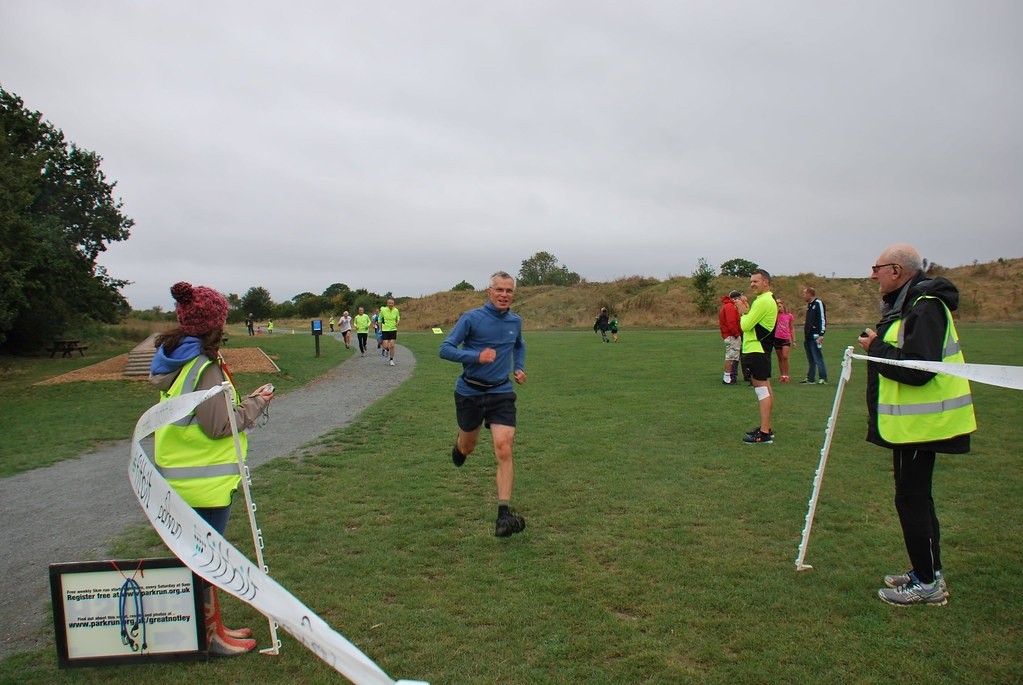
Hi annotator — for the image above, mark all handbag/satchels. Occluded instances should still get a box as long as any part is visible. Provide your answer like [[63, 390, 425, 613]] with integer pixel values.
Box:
[[461, 372, 508, 392], [607, 324, 612, 330], [594, 323, 600, 333]]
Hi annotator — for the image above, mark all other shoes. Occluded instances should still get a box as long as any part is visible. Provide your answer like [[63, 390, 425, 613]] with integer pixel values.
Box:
[[744, 377, 752, 381], [731, 378, 736, 382], [722, 380, 735, 385]]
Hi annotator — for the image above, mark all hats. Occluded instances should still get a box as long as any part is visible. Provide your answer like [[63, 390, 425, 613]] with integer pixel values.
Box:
[[729, 290, 743, 297], [170, 282, 229, 336]]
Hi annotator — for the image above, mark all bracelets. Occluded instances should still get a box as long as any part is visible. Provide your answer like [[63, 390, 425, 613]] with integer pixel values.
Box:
[[792, 340, 796, 342]]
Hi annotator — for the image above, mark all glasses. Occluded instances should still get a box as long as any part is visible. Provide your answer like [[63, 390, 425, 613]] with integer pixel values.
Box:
[[871, 264, 902, 273]]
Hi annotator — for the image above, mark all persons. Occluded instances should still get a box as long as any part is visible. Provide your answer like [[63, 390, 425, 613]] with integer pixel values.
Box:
[[337, 310, 352, 349], [857, 246, 977, 606], [372, 308, 389, 357], [353, 306, 371, 357], [609, 314, 618, 342], [257, 327, 262, 336], [266, 318, 273, 336], [717, 290, 751, 385], [437, 270, 527, 538], [147, 283, 273, 655], [595, 306, 609, 342], [328, 314, 335, 333], [798, 287, 829, 384], [773, 298, 797, 383], [739, 270, 778, 443], [377, 298, 400, 366], [245, 313, 255, 336]]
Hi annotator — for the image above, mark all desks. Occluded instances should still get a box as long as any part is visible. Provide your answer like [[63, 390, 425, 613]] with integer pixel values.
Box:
[[50, 341, 84, 358]]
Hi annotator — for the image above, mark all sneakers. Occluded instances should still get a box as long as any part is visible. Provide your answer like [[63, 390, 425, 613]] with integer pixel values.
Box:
[[799, 378, 816, 384], [741, 433, 773, 444], [452, 434, 466, 467], [382, 350, 385, 356], [385, 350, 389, 357], [745, 428, 775, 437], [885, 569, 950, 598], [818, 378, 828, 384], [778, 377, 784, 382], [784, 377, 790, 382], [495, 513, 526, 537], [878, 580, 949, 605], [389, 360, 395, 365]]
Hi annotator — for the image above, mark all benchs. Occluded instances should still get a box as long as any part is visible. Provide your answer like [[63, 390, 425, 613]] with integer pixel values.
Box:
[[47, 347, 88, 352]]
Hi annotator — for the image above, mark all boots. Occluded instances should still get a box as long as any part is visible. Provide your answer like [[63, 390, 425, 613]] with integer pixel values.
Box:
[[201, 586, 257, 655], [223, 625, 253, 639]]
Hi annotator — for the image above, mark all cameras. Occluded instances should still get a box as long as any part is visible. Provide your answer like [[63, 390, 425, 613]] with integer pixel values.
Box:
[[264, 386, 276, 393]]
